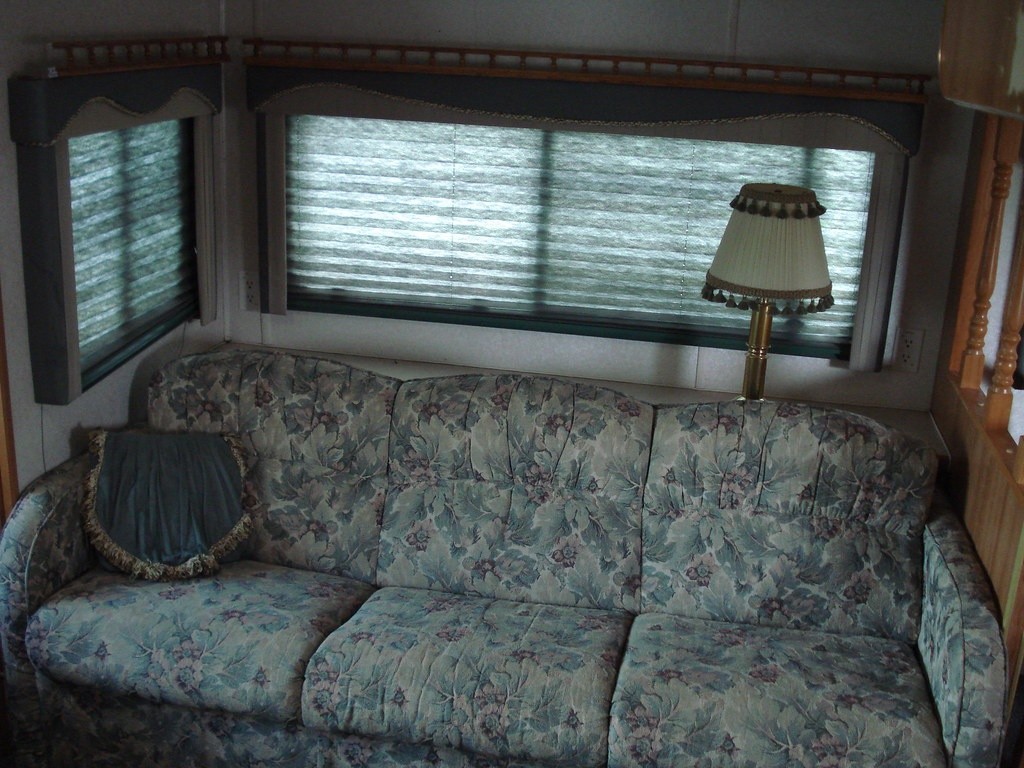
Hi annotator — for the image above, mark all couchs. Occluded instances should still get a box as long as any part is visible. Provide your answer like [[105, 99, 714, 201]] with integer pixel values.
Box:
[[0, 347, 1007, 768]]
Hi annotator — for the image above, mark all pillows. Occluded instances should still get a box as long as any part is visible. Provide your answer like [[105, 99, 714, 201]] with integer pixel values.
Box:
[[82, 429, 260, 582]]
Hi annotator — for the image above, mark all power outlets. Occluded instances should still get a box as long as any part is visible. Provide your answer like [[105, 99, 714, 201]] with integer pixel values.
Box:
[[890, 328, 924, 374]]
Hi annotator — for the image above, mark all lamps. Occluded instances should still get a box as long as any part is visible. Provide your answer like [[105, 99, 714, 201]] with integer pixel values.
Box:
[[702, 184, 833, 400]]
